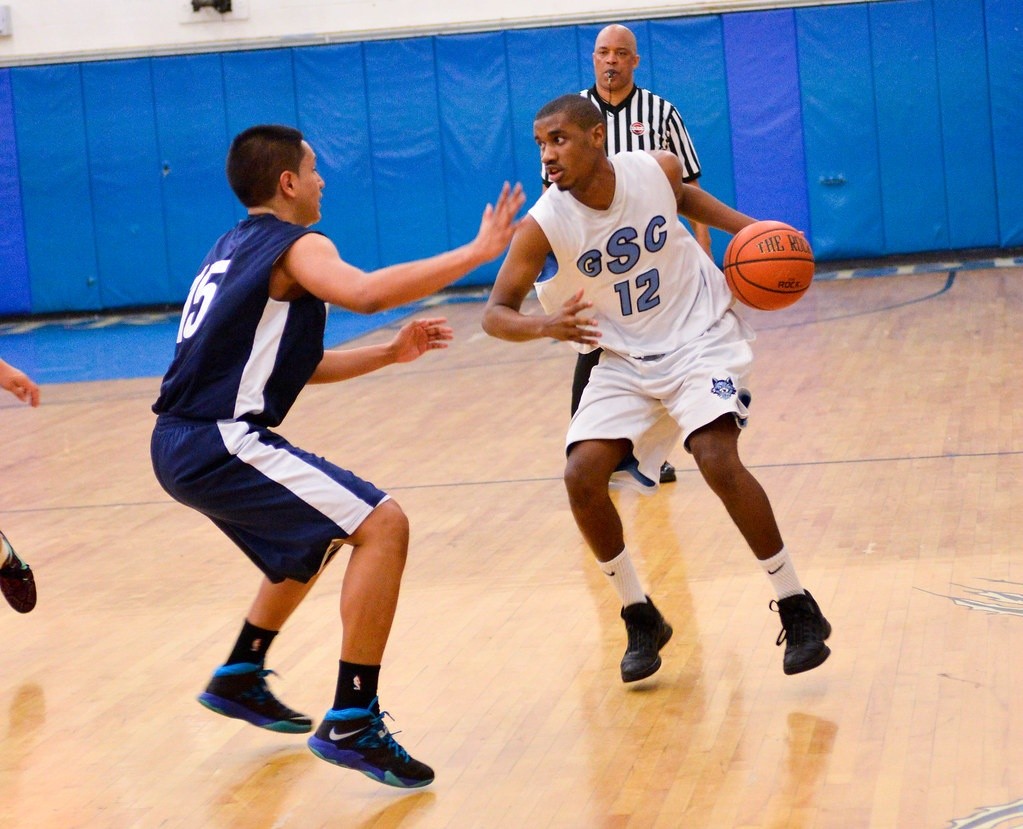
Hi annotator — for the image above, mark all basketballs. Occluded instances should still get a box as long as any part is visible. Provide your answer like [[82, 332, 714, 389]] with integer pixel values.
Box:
[[723, 220, 814, 311]]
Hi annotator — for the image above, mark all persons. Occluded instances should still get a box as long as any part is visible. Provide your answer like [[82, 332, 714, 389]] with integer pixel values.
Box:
[[481, 93, 833, 684], [154, 126, 524, 793], [0, 356, 40, 614], [540, 22, 715, 486]]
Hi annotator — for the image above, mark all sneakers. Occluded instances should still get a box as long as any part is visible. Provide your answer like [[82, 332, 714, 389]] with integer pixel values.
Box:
[[0, 531, 37, 614], [777, 589, 832, 674], [307, 696, 435, 788], [620, 595, 672, 683], [197, 658, 312, 735]]
